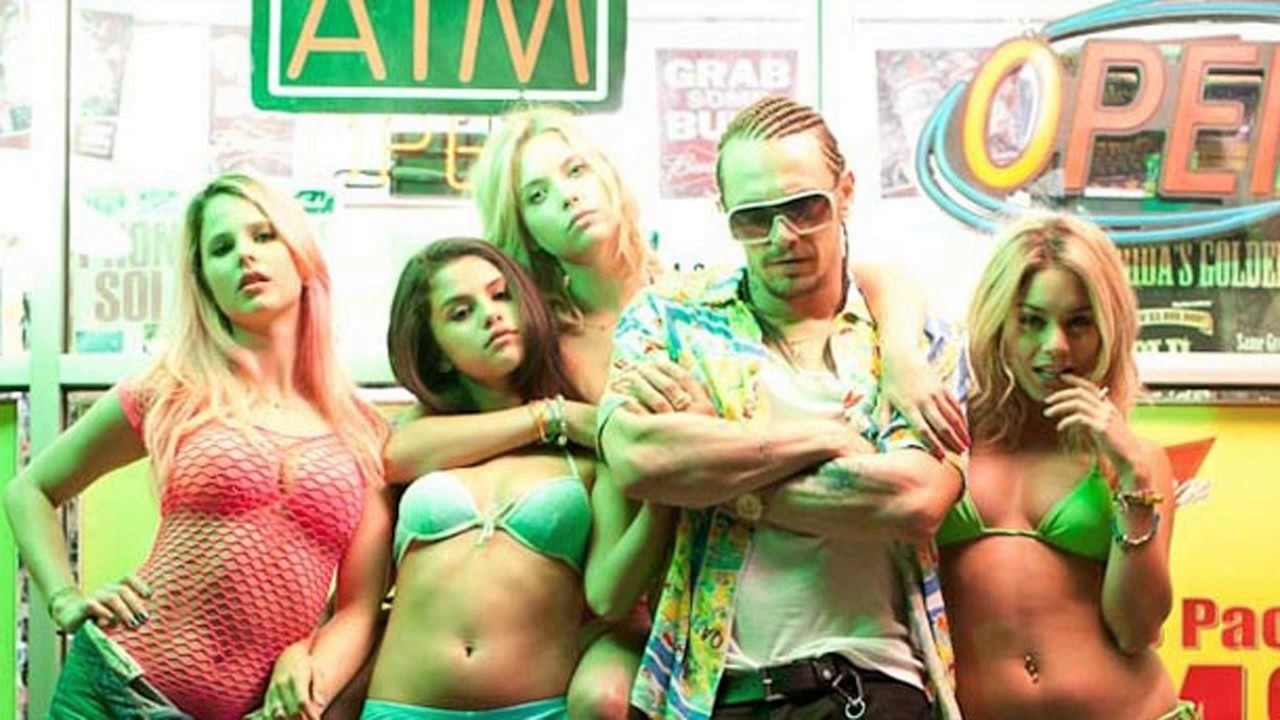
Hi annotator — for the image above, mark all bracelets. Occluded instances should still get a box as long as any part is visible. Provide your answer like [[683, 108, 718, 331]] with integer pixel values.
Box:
[[553, 394, 569, 446], [1115, 489, 1164, 509], [47, 585, 82, 618], [537, 398, 558, 442], [1112, 513, 1162, 549], [526, 399, 545, 443]]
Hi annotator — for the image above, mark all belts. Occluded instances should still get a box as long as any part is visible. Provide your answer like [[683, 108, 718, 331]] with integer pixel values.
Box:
[[717, 653, 884, 706]]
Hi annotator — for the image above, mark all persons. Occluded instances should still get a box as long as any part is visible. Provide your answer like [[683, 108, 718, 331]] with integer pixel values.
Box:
[[263, 98, 1197, 720], [2, 173, 402, 720]]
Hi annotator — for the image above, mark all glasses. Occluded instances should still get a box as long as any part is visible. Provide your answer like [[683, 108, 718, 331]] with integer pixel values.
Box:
[[729, 188, 835, 244]]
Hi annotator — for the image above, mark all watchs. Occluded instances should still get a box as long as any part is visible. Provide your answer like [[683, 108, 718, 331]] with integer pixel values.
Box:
[[737, 492, 767, 530]]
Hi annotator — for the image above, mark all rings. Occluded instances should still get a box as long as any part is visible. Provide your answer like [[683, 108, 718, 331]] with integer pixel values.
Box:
[[1098, 386, 1107, 398]]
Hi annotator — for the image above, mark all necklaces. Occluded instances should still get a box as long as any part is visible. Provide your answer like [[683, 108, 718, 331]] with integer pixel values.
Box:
[[787, 325, 823, 361], [250, 383, 294, 411], [586, 320, 617, 333]]
